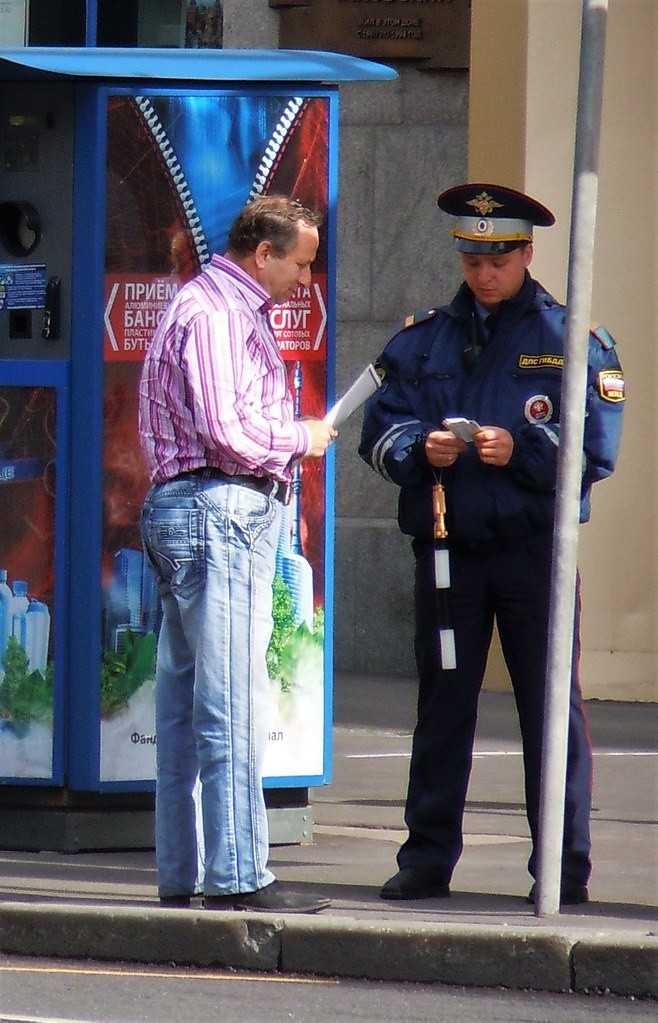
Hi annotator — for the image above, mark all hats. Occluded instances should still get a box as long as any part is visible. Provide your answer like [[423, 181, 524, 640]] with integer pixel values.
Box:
[[438, 183, 556, 255]]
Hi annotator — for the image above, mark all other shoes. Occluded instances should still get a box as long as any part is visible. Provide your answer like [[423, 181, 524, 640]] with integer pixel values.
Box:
[[528, 883, 588, 904], [380, 869, 450, 900]]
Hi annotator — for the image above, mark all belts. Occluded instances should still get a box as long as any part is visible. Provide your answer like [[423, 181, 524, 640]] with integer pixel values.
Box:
[[181, 466, 292, 506]]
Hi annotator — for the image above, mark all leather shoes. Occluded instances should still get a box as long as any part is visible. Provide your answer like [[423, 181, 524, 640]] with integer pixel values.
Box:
[[206, 880, 331, 912], [159, 891, 206, 908]]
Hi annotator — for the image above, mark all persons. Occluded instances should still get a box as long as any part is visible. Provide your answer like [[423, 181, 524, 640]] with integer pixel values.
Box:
[[357, 181, 626, 906], [139, 195, 337, 914]]
